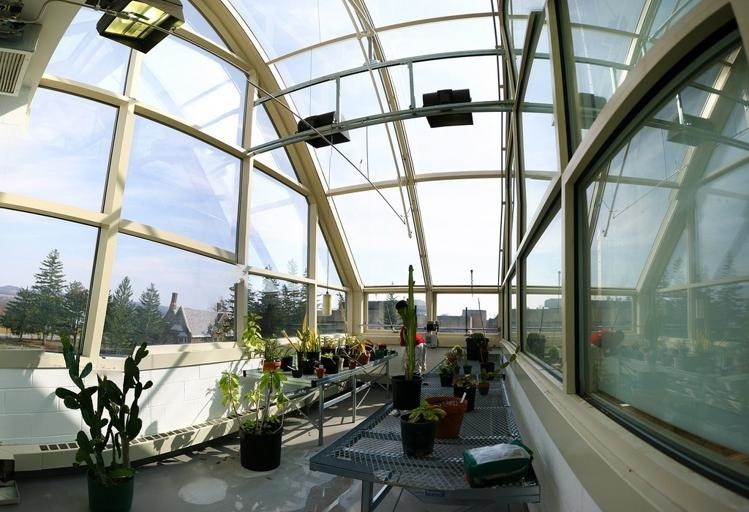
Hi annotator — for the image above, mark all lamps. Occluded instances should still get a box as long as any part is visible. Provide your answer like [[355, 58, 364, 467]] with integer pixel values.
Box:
[[423, 90, 474, 128], [297, 111, 351, 148], [322, 204, 332, 318], [96, 0, 185, 54]]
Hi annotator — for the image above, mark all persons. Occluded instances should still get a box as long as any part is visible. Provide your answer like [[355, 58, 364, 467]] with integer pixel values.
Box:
[[400, 326, 408, 373], [413, 332, 428, 376], [591, 326, 626, 359]]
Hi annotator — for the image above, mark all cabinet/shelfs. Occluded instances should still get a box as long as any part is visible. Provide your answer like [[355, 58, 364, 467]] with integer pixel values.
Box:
[[310, 352, 541, 512]]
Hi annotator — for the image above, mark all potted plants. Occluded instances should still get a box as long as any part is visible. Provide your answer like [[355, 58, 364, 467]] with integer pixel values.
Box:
[[669, 340, 691, 369], [240, 311, 388, 380], [390, 264, 424, 410], [526, 333, 560, 359], [441, 333, 497, 411], [219, 368, 289, 472], [56, 334, 154, 512], [426, 395, 468, 440], [399, 402, 446, 458]]
